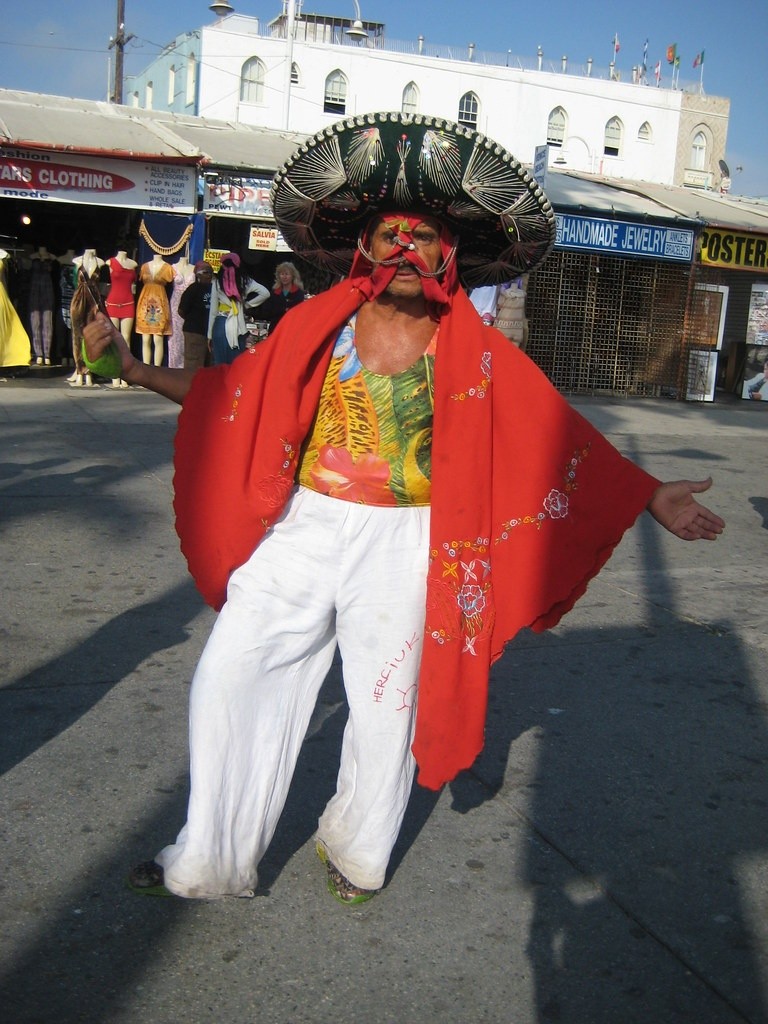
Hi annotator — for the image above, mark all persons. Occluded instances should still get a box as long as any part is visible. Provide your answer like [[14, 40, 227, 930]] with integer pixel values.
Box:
[[742, 361, 768, 401], [68, 249, 196, 388], [0, 246, 79, 368], [83, 206, 726, 905], [268, 262, 305, 336], [493, 283, 528, 346], [178, 261, 213, 371], [207, 253, 270, 367]]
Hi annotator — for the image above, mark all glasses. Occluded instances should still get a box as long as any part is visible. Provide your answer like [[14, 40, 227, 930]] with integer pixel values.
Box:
[[197, 270, 214, 275]]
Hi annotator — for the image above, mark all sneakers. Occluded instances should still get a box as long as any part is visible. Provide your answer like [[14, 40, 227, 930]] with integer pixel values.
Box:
[[316, 834, 374, 904], [129, 860, 178, 897]]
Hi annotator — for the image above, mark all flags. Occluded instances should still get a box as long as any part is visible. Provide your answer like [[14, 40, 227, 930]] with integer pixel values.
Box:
[[611, 33, 704, 81]]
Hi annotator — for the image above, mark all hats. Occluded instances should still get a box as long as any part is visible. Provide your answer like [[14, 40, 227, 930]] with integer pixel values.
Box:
[[482, 313, 495, 322], [268, 110, 557, 290]]
